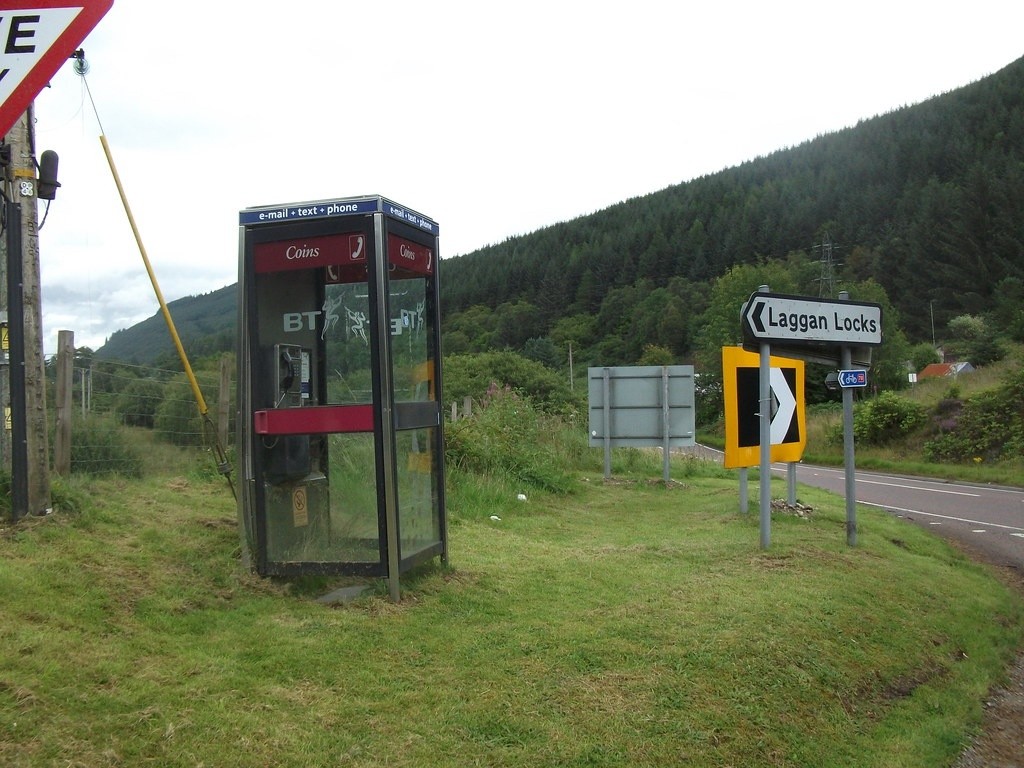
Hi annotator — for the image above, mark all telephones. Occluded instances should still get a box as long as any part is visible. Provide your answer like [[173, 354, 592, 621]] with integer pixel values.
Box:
[[272, 342, 303, 408]]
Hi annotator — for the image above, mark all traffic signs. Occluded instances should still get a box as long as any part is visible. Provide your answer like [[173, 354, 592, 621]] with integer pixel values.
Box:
[[838, 368, 868, 387], [742, 291, 885, 346]]
